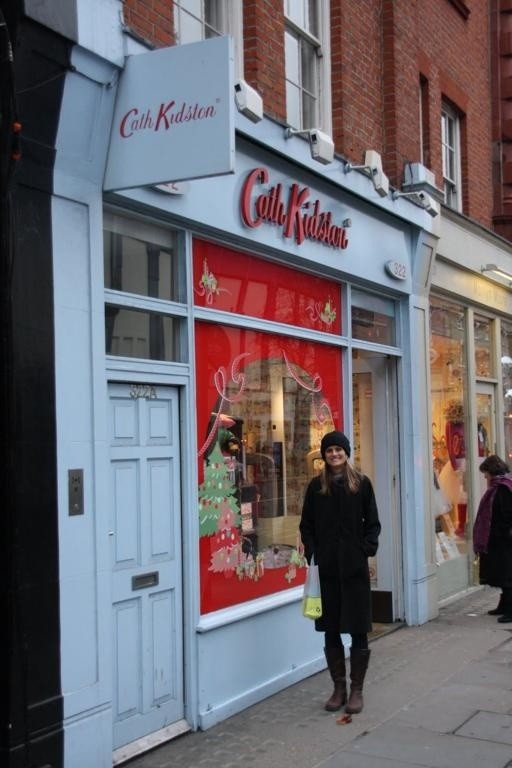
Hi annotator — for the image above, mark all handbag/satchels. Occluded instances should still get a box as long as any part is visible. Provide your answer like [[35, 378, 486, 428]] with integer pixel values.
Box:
[[303, 565, 323, 619]]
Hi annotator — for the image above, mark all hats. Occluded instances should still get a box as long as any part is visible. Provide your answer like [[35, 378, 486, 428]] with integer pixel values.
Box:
[[321, 431, 350, 458]]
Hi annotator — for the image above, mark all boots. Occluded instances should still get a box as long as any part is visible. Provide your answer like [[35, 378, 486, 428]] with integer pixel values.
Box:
[[346, 648, 370, 713], [488, 594, 511, 622], [323, 646, 346, 711]]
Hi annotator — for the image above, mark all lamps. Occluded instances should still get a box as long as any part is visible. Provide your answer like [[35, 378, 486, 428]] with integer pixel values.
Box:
[[234, 80, 264, 124], [481, 264, 512, 287], [344, 162, 389, 197], [284, 127, 335, 165], [392, 189, 438, 217]]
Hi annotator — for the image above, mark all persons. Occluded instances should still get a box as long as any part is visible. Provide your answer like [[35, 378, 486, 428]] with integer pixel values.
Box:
[[473, 455, 512, 623], [445, 395, 484, 538], [299, 431, 380, 714]]
